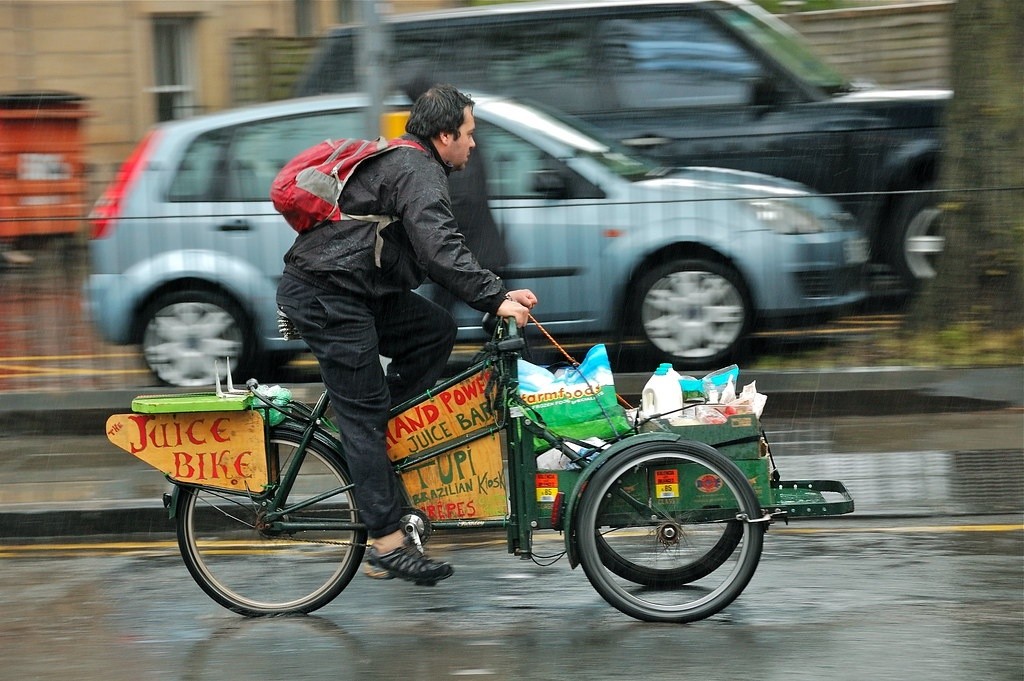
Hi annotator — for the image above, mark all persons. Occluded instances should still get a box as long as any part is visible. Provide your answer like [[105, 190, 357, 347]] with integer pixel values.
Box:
[[407, 78, 507, 388], [275, 85, 537, 582]]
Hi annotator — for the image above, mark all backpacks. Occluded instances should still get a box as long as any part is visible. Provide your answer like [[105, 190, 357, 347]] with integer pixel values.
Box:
[[271, 136, 429, 268]]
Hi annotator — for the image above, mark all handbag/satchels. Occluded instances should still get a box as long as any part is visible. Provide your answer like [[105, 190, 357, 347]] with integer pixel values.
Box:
[[517, 344, 636, 451], [678, 363, 739, 402]]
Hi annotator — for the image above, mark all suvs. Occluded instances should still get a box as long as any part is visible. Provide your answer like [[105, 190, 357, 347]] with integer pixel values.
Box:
[[79, 86, 864, 399], [246, 2, 955, 304]]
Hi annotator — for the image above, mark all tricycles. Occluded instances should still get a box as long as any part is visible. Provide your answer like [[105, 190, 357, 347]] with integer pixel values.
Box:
[[106, 299, 851, 626]]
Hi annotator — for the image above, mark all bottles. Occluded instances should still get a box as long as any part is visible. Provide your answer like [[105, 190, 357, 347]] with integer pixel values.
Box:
[[660, 363, 681, 379], [656, 367, 683, 404], [642, 371, 683, 421]]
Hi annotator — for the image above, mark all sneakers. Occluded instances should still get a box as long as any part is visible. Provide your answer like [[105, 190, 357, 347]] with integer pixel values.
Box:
[[363, 534, 454, 581]]
